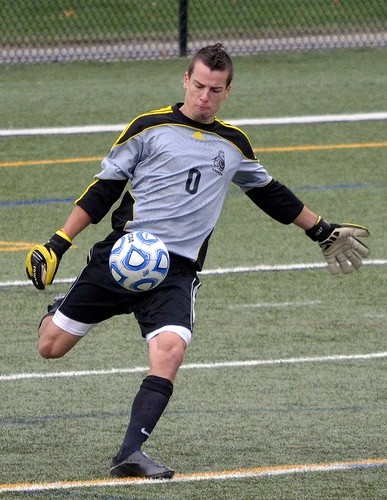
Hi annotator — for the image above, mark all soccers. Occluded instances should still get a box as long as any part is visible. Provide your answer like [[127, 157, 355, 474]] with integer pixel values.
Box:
[[109, 231, 169, 291]]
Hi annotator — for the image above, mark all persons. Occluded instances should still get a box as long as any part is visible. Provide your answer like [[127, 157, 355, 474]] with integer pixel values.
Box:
[[25, 42, 370, 479]]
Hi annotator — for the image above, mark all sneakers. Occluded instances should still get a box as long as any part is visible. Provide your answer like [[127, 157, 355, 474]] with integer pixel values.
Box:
[[110, 451, 176, 479], [47, 292, 68, 312]]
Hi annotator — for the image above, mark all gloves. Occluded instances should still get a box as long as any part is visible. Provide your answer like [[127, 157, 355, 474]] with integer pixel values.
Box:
[[306, 216, 371, 277], [25, 231, 74, 290]]
